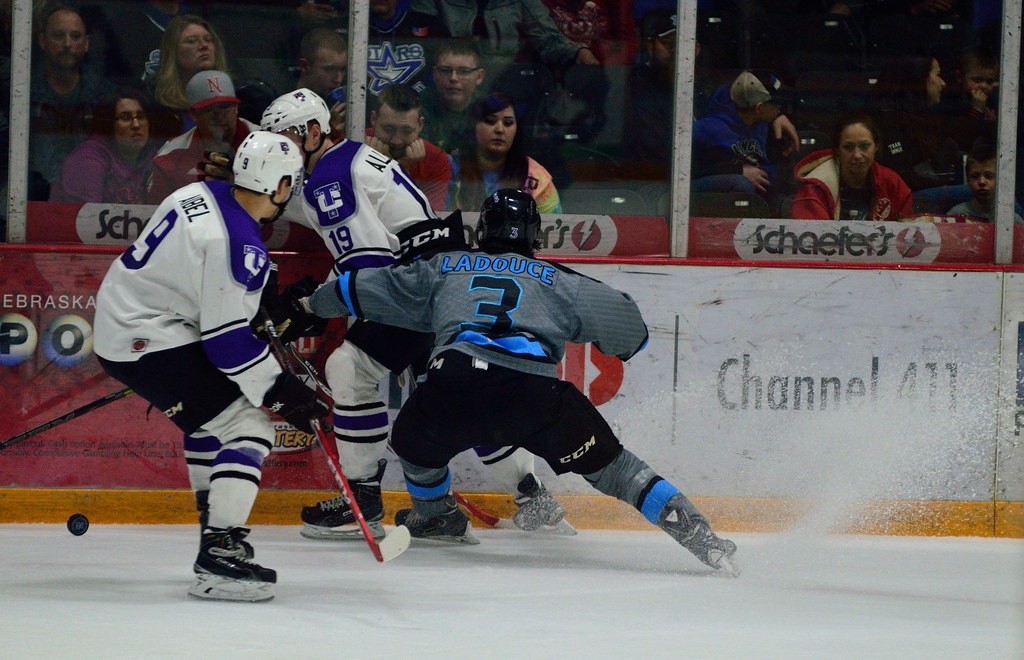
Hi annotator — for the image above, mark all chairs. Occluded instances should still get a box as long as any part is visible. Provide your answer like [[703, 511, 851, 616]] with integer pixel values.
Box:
[[554, 39, 972, 217]]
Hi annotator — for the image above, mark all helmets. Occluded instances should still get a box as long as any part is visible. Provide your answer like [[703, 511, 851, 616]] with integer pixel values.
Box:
[[474, 187, 541, 248], [260, 87, 332, 135], [232, 131, 305, 196]]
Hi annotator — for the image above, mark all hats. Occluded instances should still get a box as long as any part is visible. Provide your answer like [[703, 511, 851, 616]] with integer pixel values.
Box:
[[730, 68, 793, 107], [640, 7, 677, 41], [185, 70, 241, 112]]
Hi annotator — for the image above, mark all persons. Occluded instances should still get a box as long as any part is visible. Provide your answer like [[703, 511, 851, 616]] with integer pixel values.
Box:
[[92, 132, 334, 603], [0, 0, 1024, 225], [260, 89, 576, 537], [295, 190, 736, 578]]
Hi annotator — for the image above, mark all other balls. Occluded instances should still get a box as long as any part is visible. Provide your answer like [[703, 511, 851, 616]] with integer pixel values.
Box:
[[66, 513, 89, 536]]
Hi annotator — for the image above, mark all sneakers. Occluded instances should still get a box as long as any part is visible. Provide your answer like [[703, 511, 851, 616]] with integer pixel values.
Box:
[[658, 492, 740, 577], [188, 526, 278, 601], [513, 473, 578, 536], [299, 459, 388, 541], [196, 489, 254, 560], [395, 484, 482, 545]]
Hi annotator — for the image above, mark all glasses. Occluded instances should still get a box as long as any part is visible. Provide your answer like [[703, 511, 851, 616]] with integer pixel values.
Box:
[[434, 65, 479, 75], [111, 111, 147, 123]]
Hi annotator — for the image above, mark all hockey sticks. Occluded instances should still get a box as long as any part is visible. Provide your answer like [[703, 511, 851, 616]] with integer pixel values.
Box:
[[261, 311, 413, 565], [0, 387, 136, 451], [284, 341, 578, 534]]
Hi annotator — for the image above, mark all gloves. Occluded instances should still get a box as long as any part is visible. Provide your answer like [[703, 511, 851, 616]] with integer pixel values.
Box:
[[262, 373, 330, 434], [196, 150, 234, 185], [251, 274, 329, 352]]
